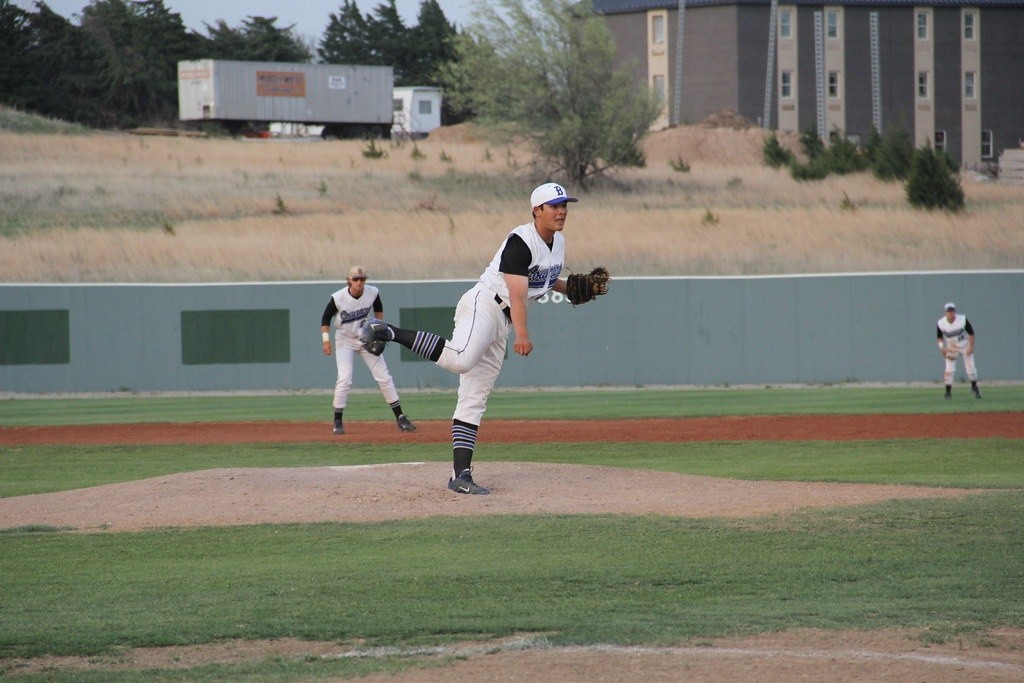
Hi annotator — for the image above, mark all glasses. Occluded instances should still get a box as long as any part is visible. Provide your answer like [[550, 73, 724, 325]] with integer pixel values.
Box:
[[350, 277, 367, 281]]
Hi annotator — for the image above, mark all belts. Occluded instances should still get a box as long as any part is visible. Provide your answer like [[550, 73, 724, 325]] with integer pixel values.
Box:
[[494, 293, 514, 320]]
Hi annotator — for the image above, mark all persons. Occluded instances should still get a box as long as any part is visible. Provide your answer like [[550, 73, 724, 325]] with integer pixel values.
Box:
[[321, 265, 416, 435], [361, 183, 610, 495], [936, 302, 982, 398]]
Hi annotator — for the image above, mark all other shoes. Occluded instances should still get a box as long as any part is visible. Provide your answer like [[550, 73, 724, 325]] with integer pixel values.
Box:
[[945, 391, 951, 401], [972, 386, 982, 399]]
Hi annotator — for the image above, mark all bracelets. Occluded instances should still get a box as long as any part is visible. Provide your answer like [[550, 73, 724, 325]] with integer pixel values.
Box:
[[938, 342, 943, 349], [322, 332, 330, 344]]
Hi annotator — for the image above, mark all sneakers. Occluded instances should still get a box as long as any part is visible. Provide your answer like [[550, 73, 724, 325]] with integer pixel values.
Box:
[[334, 420, 345, 436], [447, 466, 491, 495], [397, 416, 417, 432], [359, 317, 387, 343]]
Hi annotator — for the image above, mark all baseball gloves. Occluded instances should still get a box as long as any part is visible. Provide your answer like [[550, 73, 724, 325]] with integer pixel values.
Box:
[[943, 348, 958, 360], [363, 340, 390, 356], [565, 266, 612, 309]]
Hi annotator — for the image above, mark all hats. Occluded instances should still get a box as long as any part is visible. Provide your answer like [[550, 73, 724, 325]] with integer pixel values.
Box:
[[349, 264, 369, 279], [944, 303, 955, 312], [530, 180, 578, 209]]
